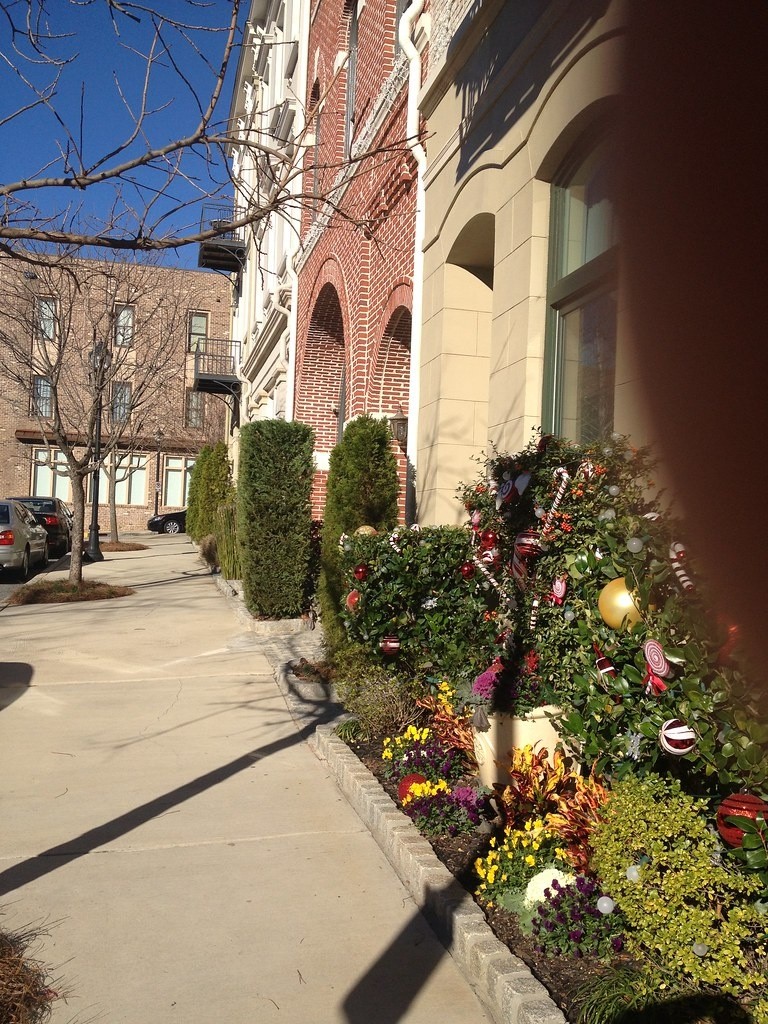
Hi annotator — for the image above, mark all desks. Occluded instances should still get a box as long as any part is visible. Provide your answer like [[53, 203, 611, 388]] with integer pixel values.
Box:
[[209, 220, 231, 239]]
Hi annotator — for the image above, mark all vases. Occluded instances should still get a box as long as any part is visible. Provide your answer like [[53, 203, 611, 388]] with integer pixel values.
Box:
[[471, 700, 581, 797]]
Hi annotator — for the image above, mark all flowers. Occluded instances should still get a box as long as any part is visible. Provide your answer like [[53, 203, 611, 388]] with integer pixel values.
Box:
[[460, 425, 620, 717]]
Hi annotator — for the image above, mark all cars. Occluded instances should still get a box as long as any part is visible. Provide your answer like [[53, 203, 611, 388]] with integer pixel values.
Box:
[[0, 499, 50, 579], [147, 508, 188, 535], [5, 494, 75, 559]]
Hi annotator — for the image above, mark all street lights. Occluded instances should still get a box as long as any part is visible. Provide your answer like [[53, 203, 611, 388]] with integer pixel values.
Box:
[[79, 339, 105, 563]]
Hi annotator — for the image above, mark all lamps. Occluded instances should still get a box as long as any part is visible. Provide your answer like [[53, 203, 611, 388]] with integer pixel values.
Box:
[[387, 404, 408, 459]]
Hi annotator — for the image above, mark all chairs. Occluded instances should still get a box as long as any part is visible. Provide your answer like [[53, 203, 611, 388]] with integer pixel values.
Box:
[[225, 230, 238, 241], [28, 504, 55, 512]]
